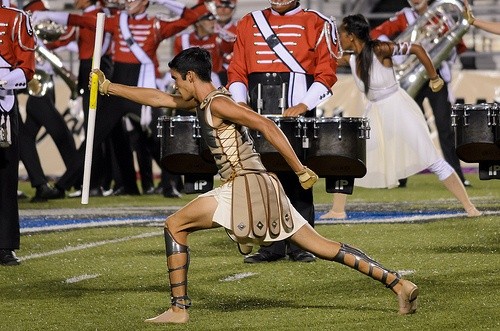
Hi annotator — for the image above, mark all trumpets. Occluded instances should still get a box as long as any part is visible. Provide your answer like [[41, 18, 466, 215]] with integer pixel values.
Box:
[[33, 45, 82, 100], [27, 69, 53, 98]]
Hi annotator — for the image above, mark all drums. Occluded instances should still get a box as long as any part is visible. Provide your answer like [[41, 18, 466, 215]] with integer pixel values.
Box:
[[247, 114, 295, 169], [156, 115, 218, 176], [295, 114, 370, 179], [450, 103, 500, 163]]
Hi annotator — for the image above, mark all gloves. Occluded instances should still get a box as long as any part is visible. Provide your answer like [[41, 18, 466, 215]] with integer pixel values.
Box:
[[31, 11, 42, 26], [88, 68, 110, 96], [150, 0, 167, 6], [429, 75, 444, 92], [295, 166, 319, 189]]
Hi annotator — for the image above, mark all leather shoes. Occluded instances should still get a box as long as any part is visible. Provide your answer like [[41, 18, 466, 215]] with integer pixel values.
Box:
[[0, 249, 20, 266], [287, 252, 316, 262], [244, 250, 286, 264]]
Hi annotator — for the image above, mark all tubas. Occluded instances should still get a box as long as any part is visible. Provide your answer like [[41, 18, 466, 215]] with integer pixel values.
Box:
[[392, 0, 471, 99]]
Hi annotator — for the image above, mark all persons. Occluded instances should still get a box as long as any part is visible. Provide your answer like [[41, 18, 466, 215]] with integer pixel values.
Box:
[[319, 12, 484, 219], [464, 0, 500, 35], [369, 0, 472, 187], [0, 0, 239, 202], [88, 47, 419, 323], [0, 0, 39, 266], [226, 0, 338, 263]]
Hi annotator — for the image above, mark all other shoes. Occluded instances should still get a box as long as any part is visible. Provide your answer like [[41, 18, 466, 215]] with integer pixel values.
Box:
[[398, 280, 418, 314], [145, 308, 189, 323], [467, 207, 482, 216], [319, 211, 346, 219]]
